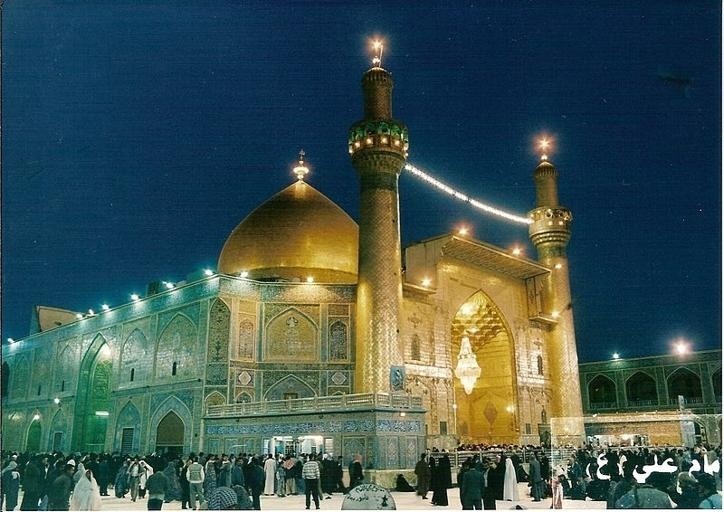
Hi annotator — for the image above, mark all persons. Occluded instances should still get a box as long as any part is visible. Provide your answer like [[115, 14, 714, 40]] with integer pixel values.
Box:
[[415, 446, 452, 506], [0, 444, 365, 510], [456, 434, 722, 509]]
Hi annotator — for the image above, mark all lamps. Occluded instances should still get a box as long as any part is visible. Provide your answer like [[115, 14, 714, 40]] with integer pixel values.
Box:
[[454, 329, 482, 396]]
